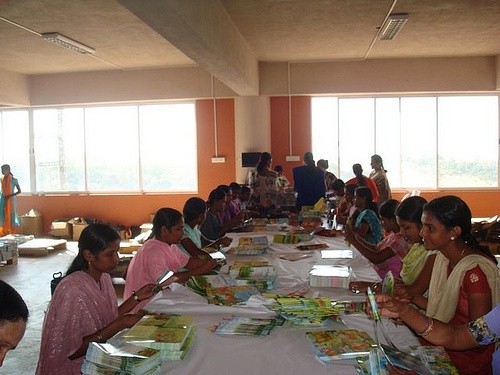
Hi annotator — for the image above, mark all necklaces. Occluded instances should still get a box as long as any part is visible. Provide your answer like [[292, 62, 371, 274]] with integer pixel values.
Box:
[[459, 246, 467, 259]]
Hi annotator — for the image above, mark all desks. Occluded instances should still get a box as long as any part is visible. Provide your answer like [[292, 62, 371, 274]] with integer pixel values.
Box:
[[143, 224, 423, 375]]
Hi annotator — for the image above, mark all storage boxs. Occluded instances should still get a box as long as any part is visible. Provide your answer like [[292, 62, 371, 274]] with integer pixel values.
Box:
[[50, 218, 89, 240], [20, 214, 44, 236]]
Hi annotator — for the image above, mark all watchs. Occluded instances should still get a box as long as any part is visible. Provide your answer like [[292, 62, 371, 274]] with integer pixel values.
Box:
[[132, 291, 141, 303]]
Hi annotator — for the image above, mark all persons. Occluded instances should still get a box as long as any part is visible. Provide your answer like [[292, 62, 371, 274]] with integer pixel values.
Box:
[[176, 198, 233, 261], [199, 180, 254, 246], [248, 152, 283, 220], [0, 277, 29, 373], [122, 205, 218, 315], [0, 163, 23, 236], [35, 223, 166, 374], [292, 151, 500, 375], [274, 166, 291, 193]]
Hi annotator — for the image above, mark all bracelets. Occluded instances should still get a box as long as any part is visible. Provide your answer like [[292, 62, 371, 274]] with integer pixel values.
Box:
[[416, 317, 434, 337]]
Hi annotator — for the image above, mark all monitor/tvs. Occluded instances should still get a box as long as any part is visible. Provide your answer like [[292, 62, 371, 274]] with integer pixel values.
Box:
[[242, 153, 261, 167]]
